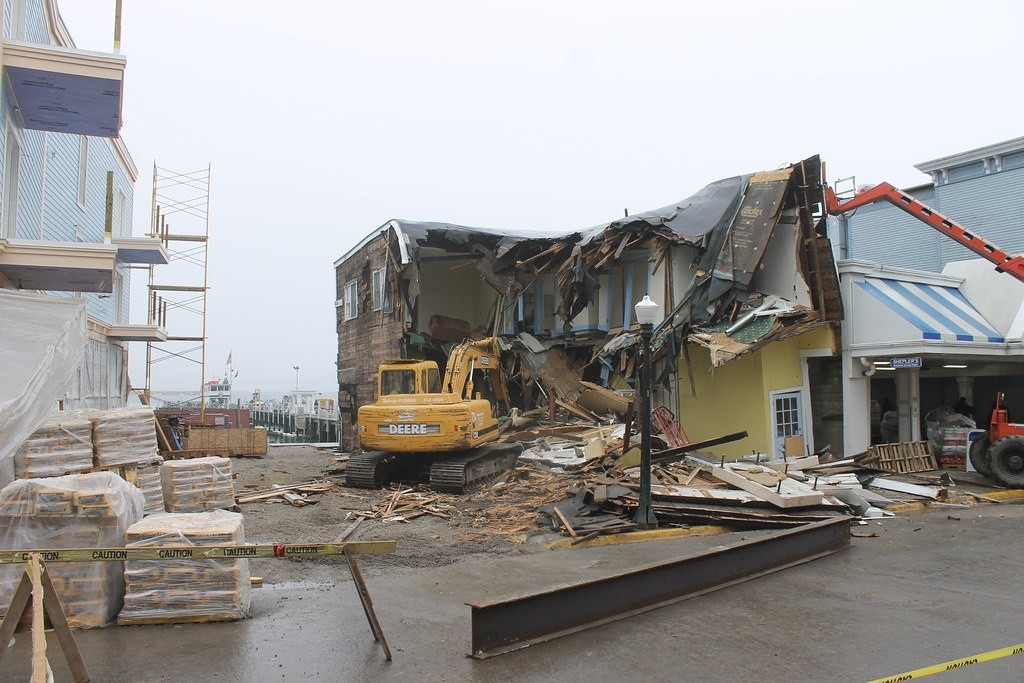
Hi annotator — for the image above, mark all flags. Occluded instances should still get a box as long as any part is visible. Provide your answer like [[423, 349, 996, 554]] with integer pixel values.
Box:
[[227, 353, 231, 365]]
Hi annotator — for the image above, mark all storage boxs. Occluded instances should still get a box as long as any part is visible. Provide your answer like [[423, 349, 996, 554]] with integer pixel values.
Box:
[[784, 433, 805, 457], [428, 314, 472, 343], [579, 380, 634, 420]]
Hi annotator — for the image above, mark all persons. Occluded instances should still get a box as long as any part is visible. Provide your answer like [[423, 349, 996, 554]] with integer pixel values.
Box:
[[314, 400, 318, 414], [954, 397, 975, 417]]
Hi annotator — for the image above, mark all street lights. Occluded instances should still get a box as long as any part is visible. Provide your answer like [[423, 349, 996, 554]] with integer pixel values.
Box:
[[633, 295, 659, 528]]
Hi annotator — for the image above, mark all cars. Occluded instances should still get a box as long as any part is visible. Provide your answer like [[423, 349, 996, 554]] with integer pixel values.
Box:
[[249, 399, 268, 410], [265, 400, 274, 408]]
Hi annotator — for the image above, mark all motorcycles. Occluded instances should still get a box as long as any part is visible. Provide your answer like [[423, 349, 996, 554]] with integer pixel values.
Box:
[[314, 398, 334, 415]]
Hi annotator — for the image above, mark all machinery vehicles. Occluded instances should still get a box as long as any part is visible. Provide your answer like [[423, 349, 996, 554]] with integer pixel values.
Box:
[[345, 336, 524, 495], [826, 183, 1024, 489]]
